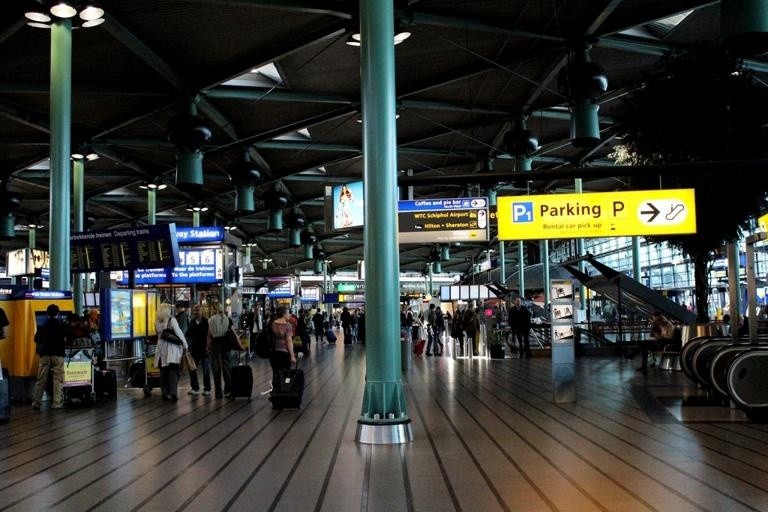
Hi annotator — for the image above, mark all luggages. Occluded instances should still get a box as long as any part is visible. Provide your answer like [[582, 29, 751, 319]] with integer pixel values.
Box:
[[413, 337, 426, 354], [233, 348, 252, 397], [325, 330, 336, 342], [272, 360, 304, 409], [0, 368, 11, 419], [344, 334, 353, 344]]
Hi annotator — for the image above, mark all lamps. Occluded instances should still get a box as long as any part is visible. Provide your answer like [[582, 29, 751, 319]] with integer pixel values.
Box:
[[258, 256, 273, 263], [345, 27, 411, 47], [138, 180, 168, 190], [186, 202, 209, 213], [242, 239, 258, 247], [21, 220, 44, 229], [224, 224, 237, 231], [70, 150, 100, 162], [24, 0, 106, 29]]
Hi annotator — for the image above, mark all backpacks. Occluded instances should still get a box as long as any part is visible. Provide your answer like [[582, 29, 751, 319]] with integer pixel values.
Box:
[[255, 318, 275, 359]]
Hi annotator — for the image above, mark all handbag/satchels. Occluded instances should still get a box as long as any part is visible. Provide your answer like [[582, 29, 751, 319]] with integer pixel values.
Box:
[[183, 348, 196, 371], [160, 316, 183, 345]]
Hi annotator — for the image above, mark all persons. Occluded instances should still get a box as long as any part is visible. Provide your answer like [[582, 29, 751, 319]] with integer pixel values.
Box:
[[242, 297, 532, 359], [153, 302, 188, 401], [175, 300, 192, 332], [267, 305, 297, 401], [206, 302, 233, 397], [718, 314, 731, 337], [185, 304, 212, 395]]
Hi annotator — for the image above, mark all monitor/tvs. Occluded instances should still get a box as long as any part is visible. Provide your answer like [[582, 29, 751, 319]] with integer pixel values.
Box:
[[399, 168, 414, 200], [36, 314, 70, 326], [70, 238, 171, 269], [324, 185, 363, 234], [332, 179, 366, 232]]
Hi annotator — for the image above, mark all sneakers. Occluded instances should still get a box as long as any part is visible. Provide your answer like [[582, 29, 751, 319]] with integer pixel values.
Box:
[[188, 389, 200, 395], [215, 392, 231, 399], [270, 391, 273, 395], [202, 391, 211, 395], [52, 402, 62, 408], [32, 403, 41, 409]]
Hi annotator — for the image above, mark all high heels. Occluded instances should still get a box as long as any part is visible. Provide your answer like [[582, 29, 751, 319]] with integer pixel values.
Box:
[[161, 394, 177, 402]]
